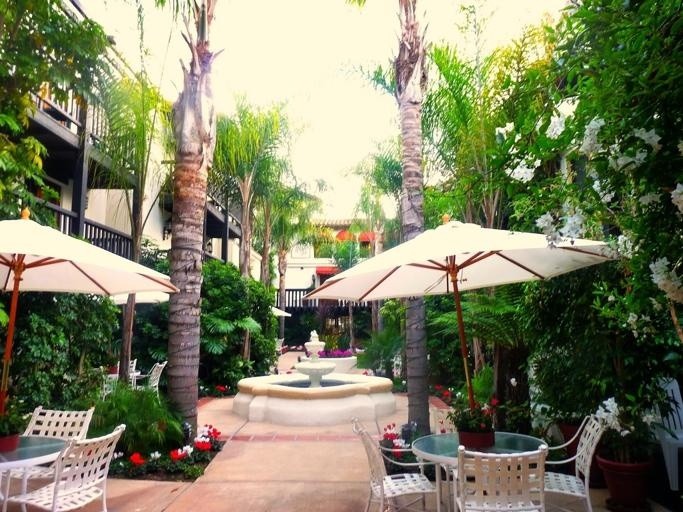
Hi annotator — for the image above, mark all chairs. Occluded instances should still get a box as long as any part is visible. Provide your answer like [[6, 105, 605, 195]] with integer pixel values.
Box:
[[0, 405, 127, 511], [273, 338, 287, 357], [637, 377, 683, 491], [351, 414, 609, 512], [391, 350, 403, 380], [98, 358, 170, 404]]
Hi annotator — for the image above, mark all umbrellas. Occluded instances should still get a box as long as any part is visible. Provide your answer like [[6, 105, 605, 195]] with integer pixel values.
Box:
[[268, 304, 290, 318], [0, 217, 178, 412], [52, 291, 171, 307], [302, 222, 620, 413]]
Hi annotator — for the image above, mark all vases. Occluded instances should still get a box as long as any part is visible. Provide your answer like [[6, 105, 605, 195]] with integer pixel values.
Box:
[[594, 447, 664, 511]]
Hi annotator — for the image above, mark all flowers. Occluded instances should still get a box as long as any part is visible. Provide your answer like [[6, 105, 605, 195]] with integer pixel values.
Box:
[[593, 374, 677, 458], [436, 387, 505, 432]]
